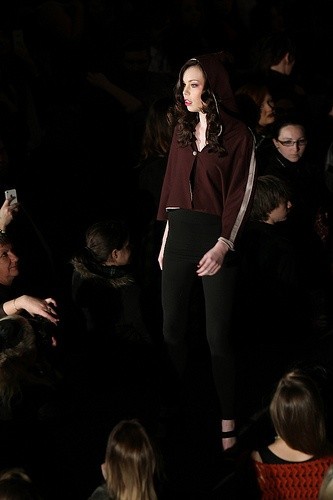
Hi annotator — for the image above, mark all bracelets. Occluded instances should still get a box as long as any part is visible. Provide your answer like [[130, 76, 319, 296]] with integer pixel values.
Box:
[[218, 236, 234, 249], [14, 299, 19, 311]]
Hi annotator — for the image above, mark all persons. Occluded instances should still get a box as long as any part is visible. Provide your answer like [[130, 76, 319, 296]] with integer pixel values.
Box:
[[156, 53, 261, 461], [70, 217, 152, 344], [0, 197, 59, 500], [87, 421, 157, 500], [251, 368, 333, 484], [0, 0, 333, 303]]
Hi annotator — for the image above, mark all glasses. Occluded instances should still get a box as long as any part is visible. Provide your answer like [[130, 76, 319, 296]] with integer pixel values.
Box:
[[275, 138, 307, 145]]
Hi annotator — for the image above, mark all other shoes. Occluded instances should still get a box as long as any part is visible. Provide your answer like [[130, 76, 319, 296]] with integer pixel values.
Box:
[[219, 419, 237, 455]]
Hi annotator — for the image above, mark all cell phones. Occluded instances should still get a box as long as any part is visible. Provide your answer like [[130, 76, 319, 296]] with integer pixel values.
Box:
[[5, 189, 18, 211]]
